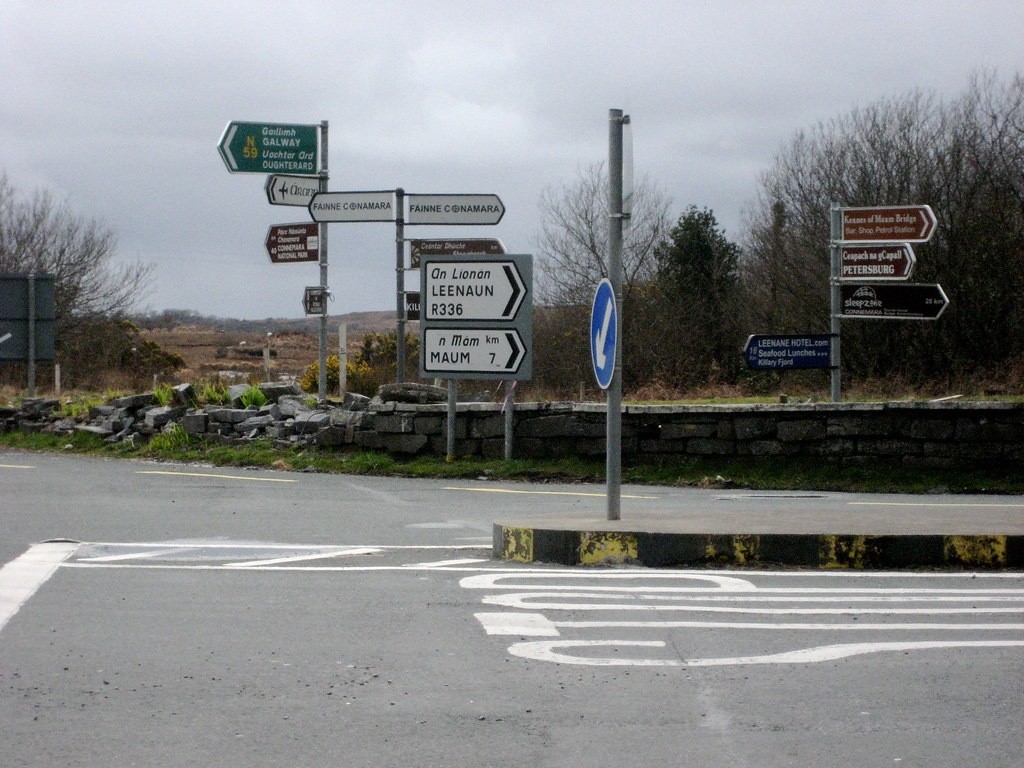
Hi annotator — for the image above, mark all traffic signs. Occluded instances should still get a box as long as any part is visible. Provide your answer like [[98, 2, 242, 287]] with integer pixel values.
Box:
[[737, 334, 830, 371], [263, 222, 322, 263], [840, 203, 937, 242], [217, 121, 321, 176], [840, 243, 917, 281], [423, 260, 529, 321], [423, 328, 527, 372], [839, 283, 950, 331], [263, 174, 323, 207]]
